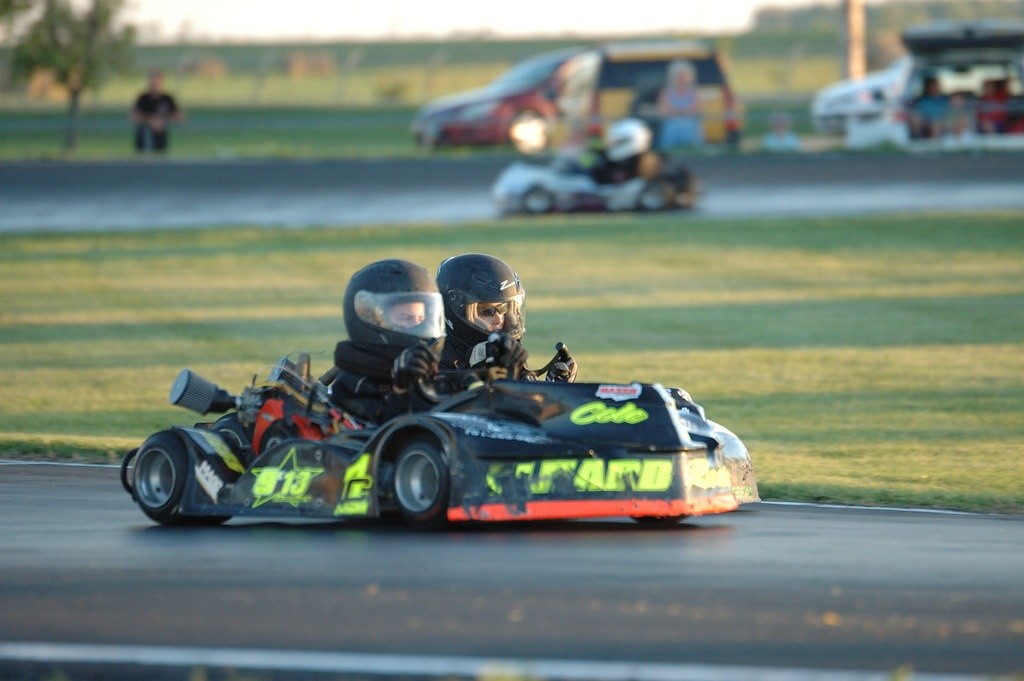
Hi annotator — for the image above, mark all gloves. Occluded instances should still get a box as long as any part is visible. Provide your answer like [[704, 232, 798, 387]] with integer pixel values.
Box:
[[484, 333, 528, 370], [391, 342, 437, 387], [545, 357, 577, 383]]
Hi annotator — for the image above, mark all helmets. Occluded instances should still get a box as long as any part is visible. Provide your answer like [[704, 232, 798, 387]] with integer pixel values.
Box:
[[436, 252, 525, 349], [603, 119, 650, 160], [343, 257, 446, 360]]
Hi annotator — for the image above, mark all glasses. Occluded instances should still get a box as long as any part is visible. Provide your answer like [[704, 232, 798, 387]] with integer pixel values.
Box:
[[477, 303, 508, 317]]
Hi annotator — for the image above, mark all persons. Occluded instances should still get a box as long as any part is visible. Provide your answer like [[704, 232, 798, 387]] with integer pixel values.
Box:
[[910, 78, 951, 137], [326, 256, 444, 427], [436, 254, 577, 383], [975, 78, 1008, 132], [657, 59, 704, 147], [130, 63, 186, 154], [580, 119, 658, 183]]
